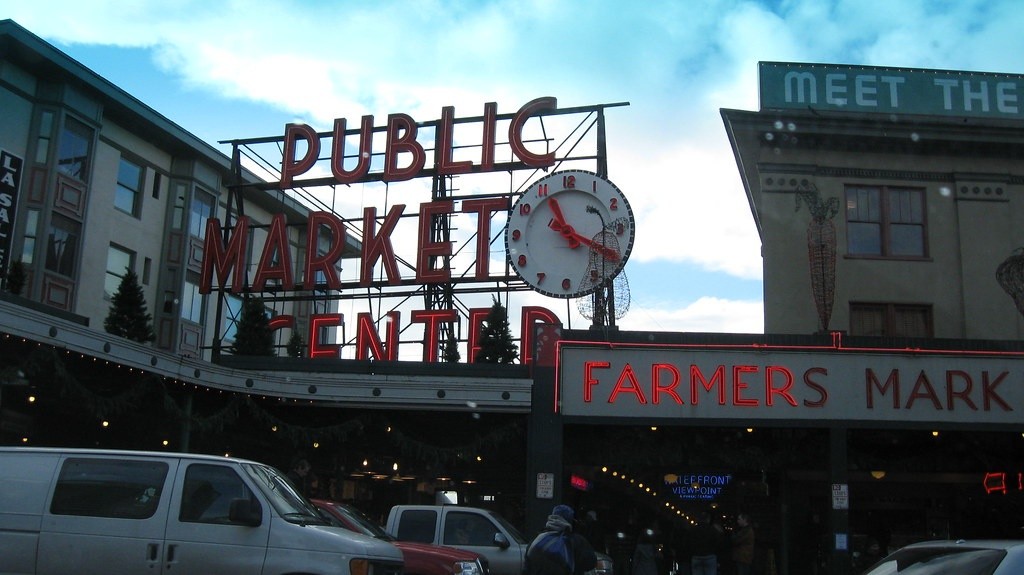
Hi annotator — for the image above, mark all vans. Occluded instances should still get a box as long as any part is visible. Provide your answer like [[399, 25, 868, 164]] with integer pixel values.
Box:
[[0, 446, 404, 575]]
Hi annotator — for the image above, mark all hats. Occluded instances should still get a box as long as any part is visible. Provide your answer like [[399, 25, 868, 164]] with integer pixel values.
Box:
[[553, 505, 575, 521]]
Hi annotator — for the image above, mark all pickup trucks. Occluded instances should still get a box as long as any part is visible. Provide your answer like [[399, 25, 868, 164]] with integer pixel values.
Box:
[[380, 505, 613, 575]]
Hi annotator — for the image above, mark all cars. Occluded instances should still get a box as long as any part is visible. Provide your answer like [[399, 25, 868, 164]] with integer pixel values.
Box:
[[308, 498, 492, 575], [860, 540, 1024, 575]]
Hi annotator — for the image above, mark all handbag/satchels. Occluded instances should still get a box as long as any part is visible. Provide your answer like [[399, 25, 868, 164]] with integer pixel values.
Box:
[[526, 531, 576, 574]]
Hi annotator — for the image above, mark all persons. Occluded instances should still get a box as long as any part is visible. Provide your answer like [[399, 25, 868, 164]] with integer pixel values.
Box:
[[454, 519, 471, 545], [856, 536, 883, 575], [632, 512, 755, 575], [286, 459, 319, 498], [525, 505, 597, 575]]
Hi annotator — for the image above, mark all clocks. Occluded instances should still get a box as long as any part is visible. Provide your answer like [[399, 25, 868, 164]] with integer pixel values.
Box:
[[505, 168, 637, 299]]
